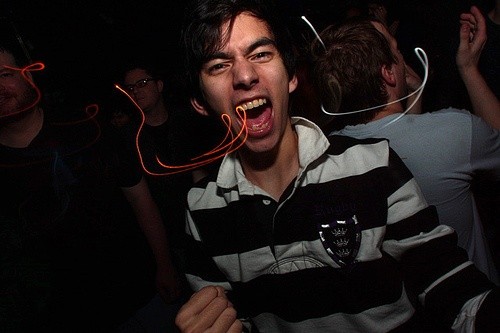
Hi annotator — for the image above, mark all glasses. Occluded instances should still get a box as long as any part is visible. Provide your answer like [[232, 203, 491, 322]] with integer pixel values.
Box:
[[120, 77, 155, 93]]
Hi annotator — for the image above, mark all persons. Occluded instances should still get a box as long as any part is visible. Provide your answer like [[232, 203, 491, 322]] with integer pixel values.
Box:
[[0, 0, 500, 333]]
